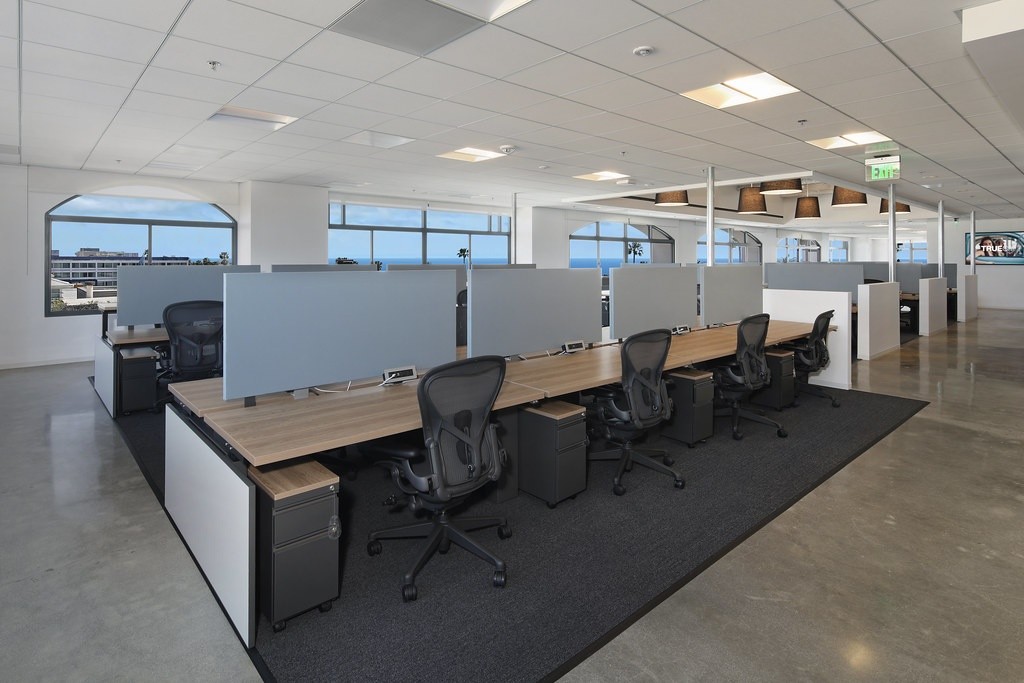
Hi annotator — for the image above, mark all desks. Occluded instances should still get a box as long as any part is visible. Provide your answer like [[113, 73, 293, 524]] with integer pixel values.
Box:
[[203, 377, 545, 473], [611, 330, 781, 364], [707, 320, 838, 343], [504, 345, 692, 398], [97, 302, 117, 339], [105, 327, 170, 351]]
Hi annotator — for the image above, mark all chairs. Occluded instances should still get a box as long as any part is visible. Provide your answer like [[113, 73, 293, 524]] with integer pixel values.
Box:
[[772, 309, 841, 408], [581, 329, 685, 496], [364, 354, 513, 604], [151, 300, 223, 414], [703, 313, 787, 440]]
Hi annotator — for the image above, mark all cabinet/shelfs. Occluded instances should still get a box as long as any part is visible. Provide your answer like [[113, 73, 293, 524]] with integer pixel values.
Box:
[[518, 402, 588, 509], [119, 347, 160, 416], [658, 370, 716, 449], [750, 348, 796, 412], [247, 461, 341, 632]]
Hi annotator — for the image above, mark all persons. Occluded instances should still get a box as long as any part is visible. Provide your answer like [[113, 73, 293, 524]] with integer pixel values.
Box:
[[965, 236, 999, 264], [995, 239, 1015, 256]]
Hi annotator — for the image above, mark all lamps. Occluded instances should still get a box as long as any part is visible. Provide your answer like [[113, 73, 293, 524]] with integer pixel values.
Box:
[[760, 179, 803, 195], [879, 199, 911, 214], [737, 183, 767, 215], [830, 186, 868, 208], [654, 190, 689, 206], [794, 181, 822, 219]]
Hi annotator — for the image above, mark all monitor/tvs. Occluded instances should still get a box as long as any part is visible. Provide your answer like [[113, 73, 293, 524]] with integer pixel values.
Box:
[[965, 231, 1024, 265]]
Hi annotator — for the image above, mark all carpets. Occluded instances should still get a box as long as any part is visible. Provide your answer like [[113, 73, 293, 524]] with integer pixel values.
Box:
[[88, 375, 931, 683]]
